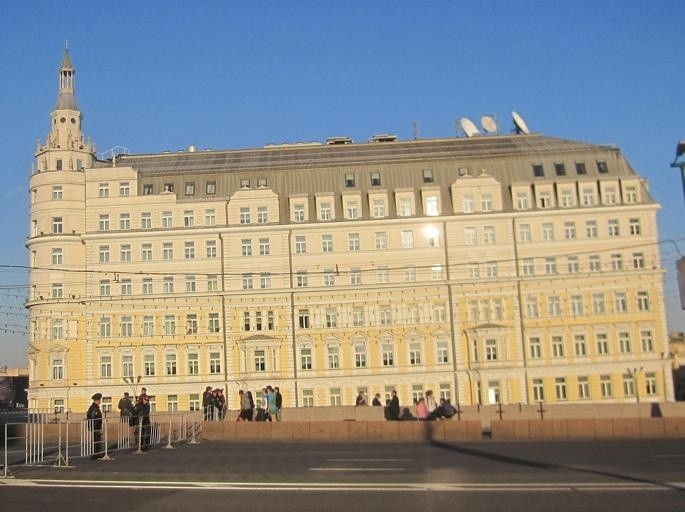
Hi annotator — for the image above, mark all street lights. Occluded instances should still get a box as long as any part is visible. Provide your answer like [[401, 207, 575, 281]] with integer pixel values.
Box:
[[123, 375, 141, 402], [626, 365, 644, 402]]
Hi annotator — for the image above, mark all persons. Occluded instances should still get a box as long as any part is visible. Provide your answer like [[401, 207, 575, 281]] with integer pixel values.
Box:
[[384, 388, 458, 421], [118, 387, 151, 452], [200, 384, 283, 424], [355, 390, 367, 406], [371, 393, 382, 406], [86, 393, 104, 459]]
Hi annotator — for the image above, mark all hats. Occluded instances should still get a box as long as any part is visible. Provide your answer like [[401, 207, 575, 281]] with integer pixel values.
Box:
[[142, 395, 152, 401], [92, 393, 102, 400], [206, 386, 212, 391]]
